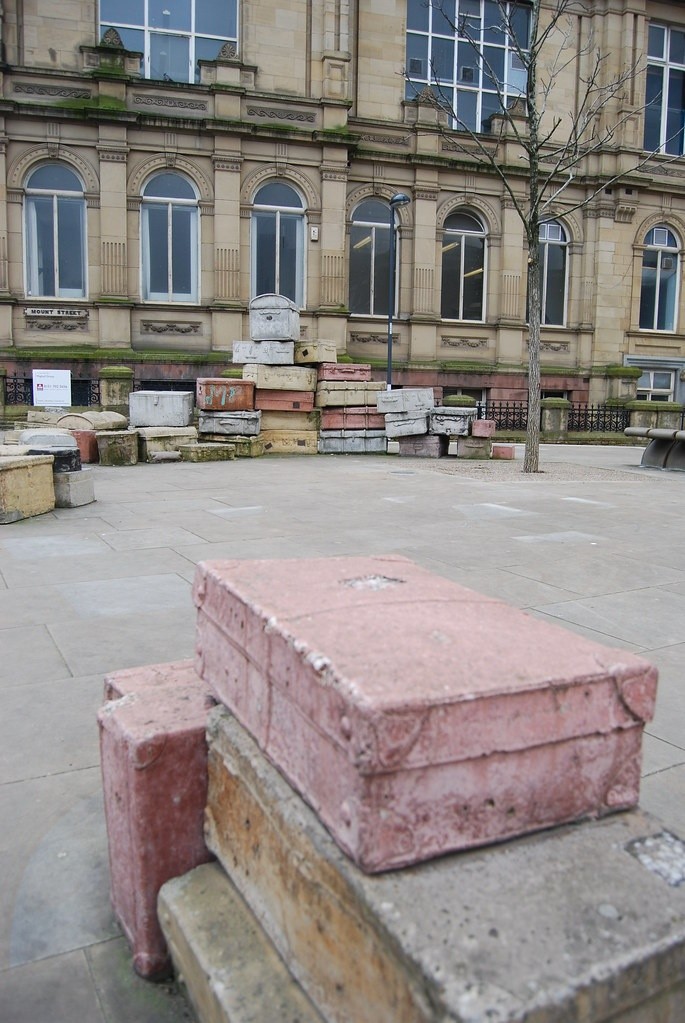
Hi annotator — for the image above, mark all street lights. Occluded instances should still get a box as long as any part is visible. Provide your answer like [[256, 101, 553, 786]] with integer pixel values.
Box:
[[387, 193, 411, 390]]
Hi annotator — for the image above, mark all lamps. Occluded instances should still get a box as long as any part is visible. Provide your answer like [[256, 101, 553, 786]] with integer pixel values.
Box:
[[442, 241, 460, 254], [352, 236, 372, 249], [464, 268, 484, 278]]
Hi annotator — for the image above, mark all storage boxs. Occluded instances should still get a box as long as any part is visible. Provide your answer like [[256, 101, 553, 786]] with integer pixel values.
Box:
[[191, 554, 659, 877], [97, 681, 214, 981], [155, 860, 320, 1023], [129, 390, 195, 426], [194, 293, 515, 460], [101, 658, 197, 706], [203, 703, 685, 1023], [0, 427, 198, 524]]
